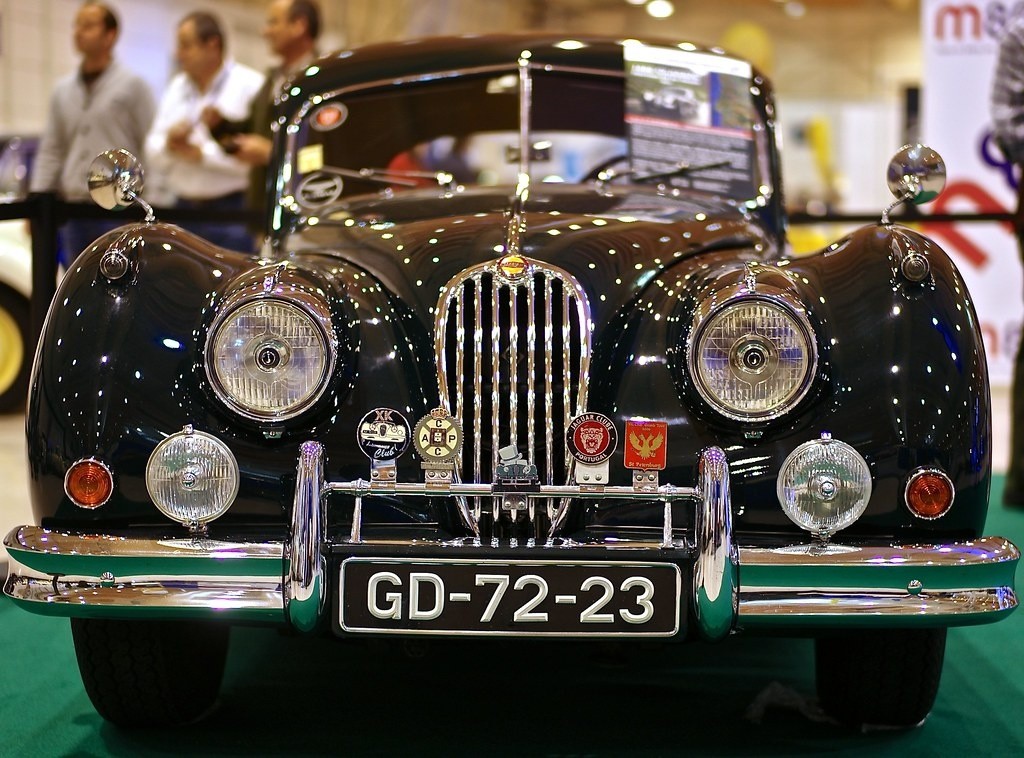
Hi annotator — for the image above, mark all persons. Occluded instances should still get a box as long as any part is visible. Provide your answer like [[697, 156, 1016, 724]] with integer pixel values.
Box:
[[143, 12, 271, 254], [989, 28, 1024, 512], [199, 0, 324, 255], [28, 1, 161, 274]]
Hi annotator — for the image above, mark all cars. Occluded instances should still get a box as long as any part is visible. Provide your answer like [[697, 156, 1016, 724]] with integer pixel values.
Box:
[[0, 30, 1021, 758], [0, 136, 69, 418]]
